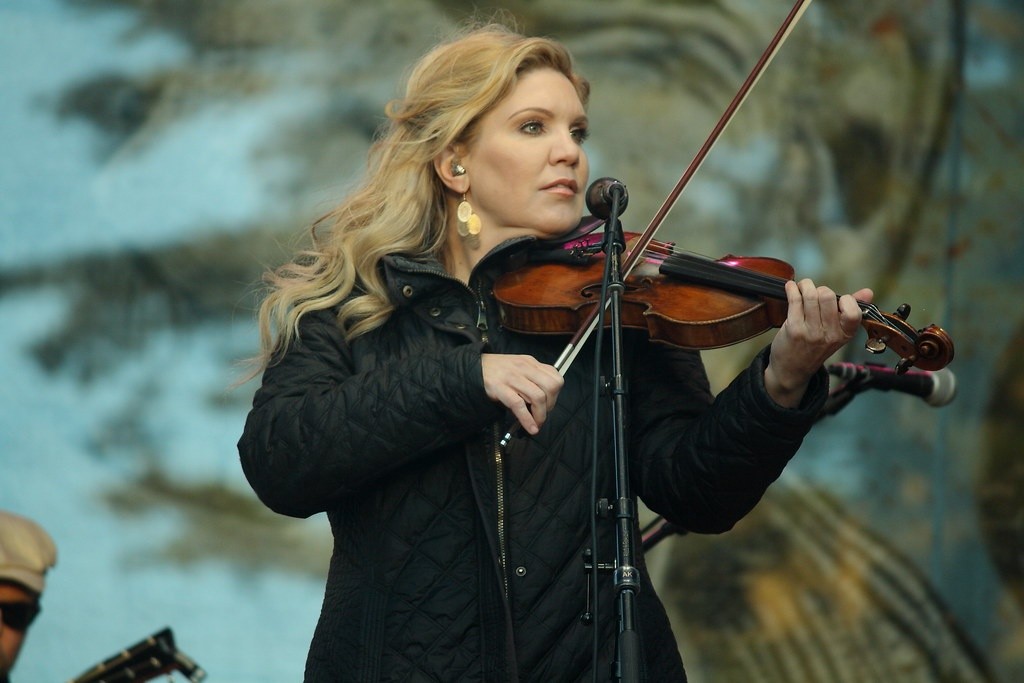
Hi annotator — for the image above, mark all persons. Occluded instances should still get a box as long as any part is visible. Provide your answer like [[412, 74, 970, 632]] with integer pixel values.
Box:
[[233, 13, 874, 683], [0, 507, 60, 683]]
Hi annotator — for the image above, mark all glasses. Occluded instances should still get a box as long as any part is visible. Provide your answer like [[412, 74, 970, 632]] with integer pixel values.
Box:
[[0, 600, 41, 632]]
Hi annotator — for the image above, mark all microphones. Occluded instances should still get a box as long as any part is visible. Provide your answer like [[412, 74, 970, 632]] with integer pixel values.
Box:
[[829, 361, 960, 408], [585, 176, 629, 220]]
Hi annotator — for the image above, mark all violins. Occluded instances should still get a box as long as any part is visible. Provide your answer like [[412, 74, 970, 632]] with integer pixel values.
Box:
[[493, 232, 956, 376]]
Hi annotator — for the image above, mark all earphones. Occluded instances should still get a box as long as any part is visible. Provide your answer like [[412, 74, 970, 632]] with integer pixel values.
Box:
[[450, 159, 465, 177]]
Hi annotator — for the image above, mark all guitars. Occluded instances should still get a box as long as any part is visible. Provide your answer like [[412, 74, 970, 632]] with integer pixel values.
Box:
[[70, 626, 178, 683]]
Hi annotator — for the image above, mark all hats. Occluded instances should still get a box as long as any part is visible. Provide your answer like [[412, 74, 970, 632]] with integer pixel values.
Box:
[[0, 511, 57, 594]]
